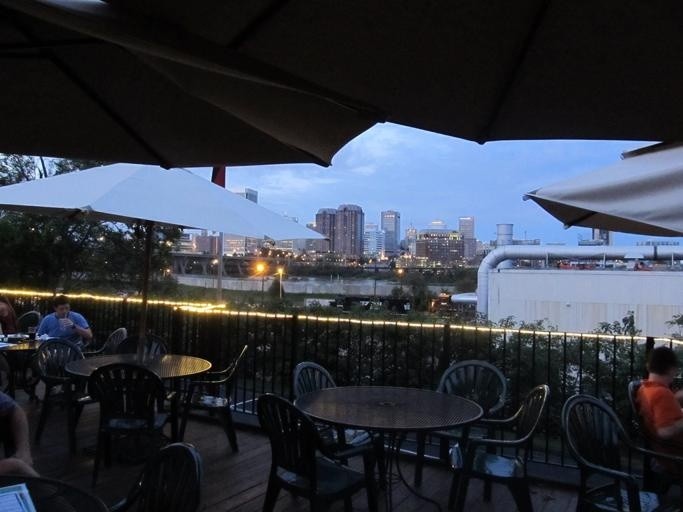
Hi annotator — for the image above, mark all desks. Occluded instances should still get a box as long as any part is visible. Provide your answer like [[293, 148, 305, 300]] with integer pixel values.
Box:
[[293, 385, 484, 510], [64, 353, 213, 445]]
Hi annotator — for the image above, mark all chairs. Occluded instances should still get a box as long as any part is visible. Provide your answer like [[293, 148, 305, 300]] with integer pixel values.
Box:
[[627, 377, 681, 511], [415, 359, 509, 496], [562, 394, 681, 511], [175, 344, 249, 452], [446, 382, 552, 509], [86, 361, 169, 488], [292, 362, 384, 511], [254, 392, 382, 510], [111, 441, 207, 511], [115, 333, 170, 424], [34, 337, 100, 452], [2, 309, 129, 405]]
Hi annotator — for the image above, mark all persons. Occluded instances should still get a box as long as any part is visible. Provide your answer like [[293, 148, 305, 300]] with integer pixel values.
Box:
[[636, 345, 683, 483], [0, 382, 41, 478], [36, 294, 93, 365], [0, 293, 22, 335]]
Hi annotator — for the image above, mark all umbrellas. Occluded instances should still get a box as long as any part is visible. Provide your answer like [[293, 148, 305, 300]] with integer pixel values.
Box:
[[0, 0, 682, 171], [521, 141, 683, 238], [0, 160, 332, 368]]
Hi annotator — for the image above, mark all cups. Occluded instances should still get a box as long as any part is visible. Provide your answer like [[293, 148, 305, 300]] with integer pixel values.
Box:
[[28, 327, 36, 341], [59, 316, 70, 332], [29, 341, 35, 349]]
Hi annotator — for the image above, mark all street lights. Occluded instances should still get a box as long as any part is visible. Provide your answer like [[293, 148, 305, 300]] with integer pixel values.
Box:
[[162, 270, 169, 277], [277, 268, 282, 298], [398, 269, 403, 287], [368, 258, 376, 273], [256, 264, 264, 305], [209, 259, 218, 288]]
